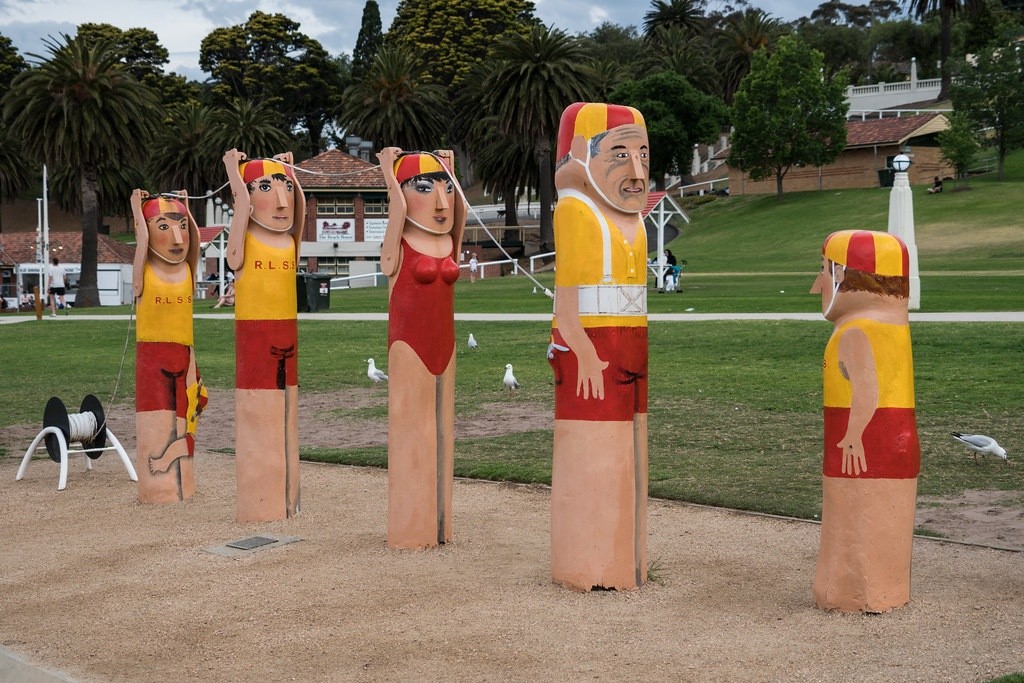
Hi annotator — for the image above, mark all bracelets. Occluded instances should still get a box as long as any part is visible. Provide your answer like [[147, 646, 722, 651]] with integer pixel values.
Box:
[[48, 289, 50, 290]]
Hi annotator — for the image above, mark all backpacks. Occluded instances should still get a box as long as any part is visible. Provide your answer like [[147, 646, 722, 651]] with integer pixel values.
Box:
[[1, 299, 7, 308]]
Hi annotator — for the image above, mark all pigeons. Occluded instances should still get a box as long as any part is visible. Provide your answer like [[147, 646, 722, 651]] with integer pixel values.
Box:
[[467, 332, 478, 353], [502, 363, 521, 398], [950, 428, 1009, 466], [366, 358, 389, 387], [532, 286, 537, 295]]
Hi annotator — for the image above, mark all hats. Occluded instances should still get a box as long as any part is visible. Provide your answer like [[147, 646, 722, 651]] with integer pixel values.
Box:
[[472, 253, 477, 257]]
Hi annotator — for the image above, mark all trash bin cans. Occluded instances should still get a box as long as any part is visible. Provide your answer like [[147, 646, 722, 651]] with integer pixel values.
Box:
[[297, 273, 334, 312], [877, 168, 895, 187]]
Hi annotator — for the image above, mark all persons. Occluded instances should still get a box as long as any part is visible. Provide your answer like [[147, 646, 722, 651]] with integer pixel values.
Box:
[[0, 291, 64, 311], [926, 176, 942, 194], [375, 148, 469, 550], [652, 249, 676, 288], [221, 149, 306, 525], [207, 269, 235, 309], [469, 252, 478, 283], [131, 188, 208, 504], [543, 103, 650, 593], [810, 229, 919, 613], [47, 257, 68, 317]]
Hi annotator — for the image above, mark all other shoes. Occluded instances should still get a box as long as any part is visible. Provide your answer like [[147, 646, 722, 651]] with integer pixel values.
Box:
[[50, 314, 56, 317], [214, 306, 220, 308], [654, 286, 657, 288], [210, 293, 214, 296]]
